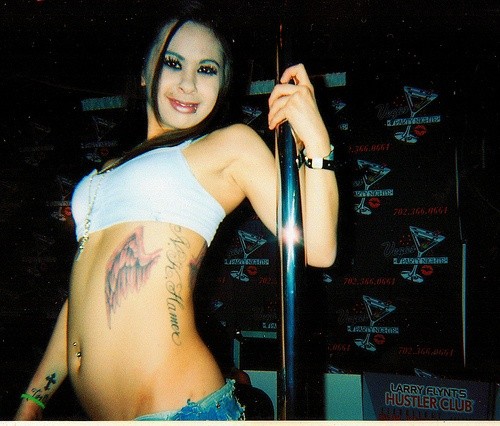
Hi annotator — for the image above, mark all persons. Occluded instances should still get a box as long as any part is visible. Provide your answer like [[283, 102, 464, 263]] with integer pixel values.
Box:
[[13, 1, 339, 422]]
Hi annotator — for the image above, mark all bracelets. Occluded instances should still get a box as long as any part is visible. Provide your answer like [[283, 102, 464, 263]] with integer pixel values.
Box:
[[297, 144, 336, 173], [20, 394, 46, 410]]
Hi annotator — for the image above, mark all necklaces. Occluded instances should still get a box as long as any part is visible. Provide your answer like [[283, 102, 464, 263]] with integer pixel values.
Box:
[[74, 155, 124, 261]]
